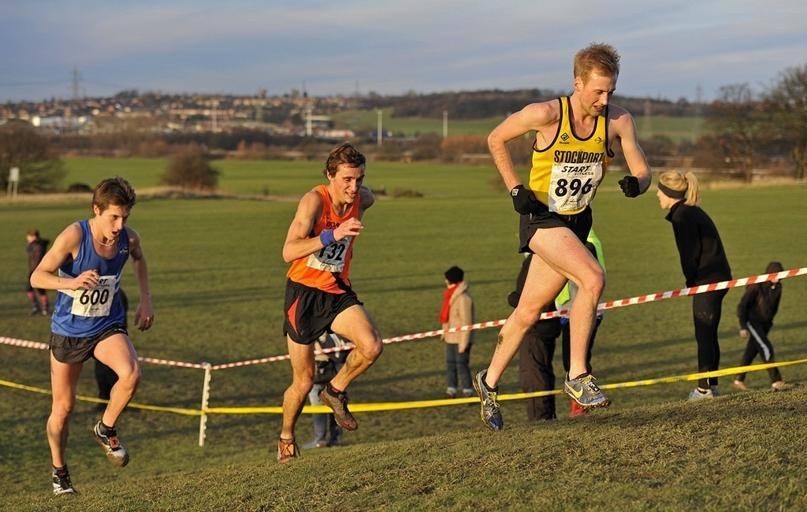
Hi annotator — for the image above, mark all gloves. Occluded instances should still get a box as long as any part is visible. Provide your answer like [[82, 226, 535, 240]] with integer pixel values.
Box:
[[510, 184, 541, 216], [619, 176, 640, 197]]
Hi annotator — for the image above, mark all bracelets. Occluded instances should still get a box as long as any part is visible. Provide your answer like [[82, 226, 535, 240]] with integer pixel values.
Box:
[[320, 229, 337, 247]]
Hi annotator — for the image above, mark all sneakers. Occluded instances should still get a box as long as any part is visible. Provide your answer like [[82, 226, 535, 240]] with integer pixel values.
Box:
[[92, 421, 129, 468], [51, 467, 77, 497], [687, 381, 788, 401], [564, 370, 612, 409], [277, 435, 297, 464], [471, 368, 504, 432], [317, 383, 358, 432]]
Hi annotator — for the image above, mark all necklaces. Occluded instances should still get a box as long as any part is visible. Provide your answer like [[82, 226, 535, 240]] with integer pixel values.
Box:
[[90, 218, 115, 247]]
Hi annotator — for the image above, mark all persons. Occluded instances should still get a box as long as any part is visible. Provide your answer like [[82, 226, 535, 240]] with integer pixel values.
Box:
[[731, 262, 788, 391], [276, 143, 383, 463], [471, 45, 652, 432], [439, 266, 476, 398], [92, 288, 128, 410], [508, 251, 560, 423], [554, 226, 607, 416], [29, 176, 154, 497], [656, 170, 733, 400], [25, 230, 50, 316], [303, 331, 351, 450]]
